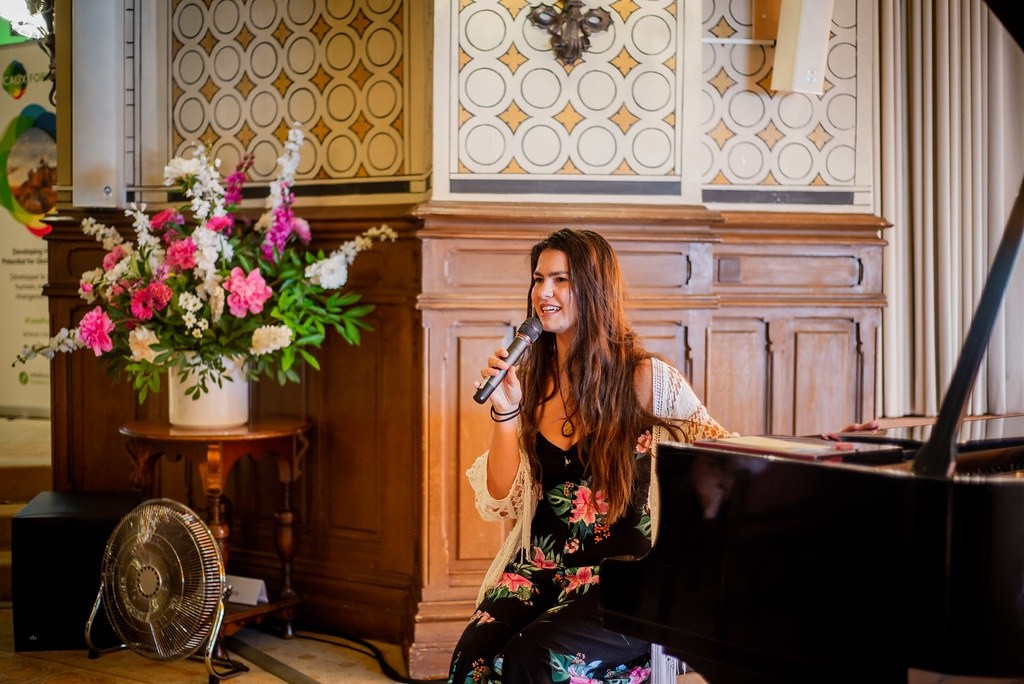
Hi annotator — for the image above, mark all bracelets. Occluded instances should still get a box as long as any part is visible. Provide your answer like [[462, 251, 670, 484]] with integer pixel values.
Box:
[[490, 402, 522, 422]]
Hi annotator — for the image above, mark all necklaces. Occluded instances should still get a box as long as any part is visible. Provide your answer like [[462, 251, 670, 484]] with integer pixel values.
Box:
[[556, 350, 581, 436]]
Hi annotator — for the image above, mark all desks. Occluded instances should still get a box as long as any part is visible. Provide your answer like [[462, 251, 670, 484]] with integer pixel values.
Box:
[[121, 410, 315, 657]]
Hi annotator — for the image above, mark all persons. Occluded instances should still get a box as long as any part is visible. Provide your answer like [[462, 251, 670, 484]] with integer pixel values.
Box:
[[449, 227, 881, 684]]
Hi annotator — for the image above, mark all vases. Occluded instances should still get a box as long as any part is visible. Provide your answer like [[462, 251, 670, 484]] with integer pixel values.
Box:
[[167, 347, 254, 430]]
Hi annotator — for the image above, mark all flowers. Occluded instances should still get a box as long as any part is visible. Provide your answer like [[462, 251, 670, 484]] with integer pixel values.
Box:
[[9, 113, 376, 400]]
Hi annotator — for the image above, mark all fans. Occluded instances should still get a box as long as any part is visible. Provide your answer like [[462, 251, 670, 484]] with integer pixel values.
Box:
[[85, 494, 251, 684]]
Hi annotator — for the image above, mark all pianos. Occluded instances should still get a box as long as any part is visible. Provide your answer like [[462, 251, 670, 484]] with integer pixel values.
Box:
[[599, 1, 1024, 683]]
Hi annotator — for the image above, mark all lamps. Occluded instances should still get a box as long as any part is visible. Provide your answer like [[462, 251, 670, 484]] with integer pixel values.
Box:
[[749, 0, 833, 95]]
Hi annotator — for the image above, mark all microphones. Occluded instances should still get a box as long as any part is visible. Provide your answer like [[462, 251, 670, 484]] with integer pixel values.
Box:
[[473, 316, 543, 404]]
